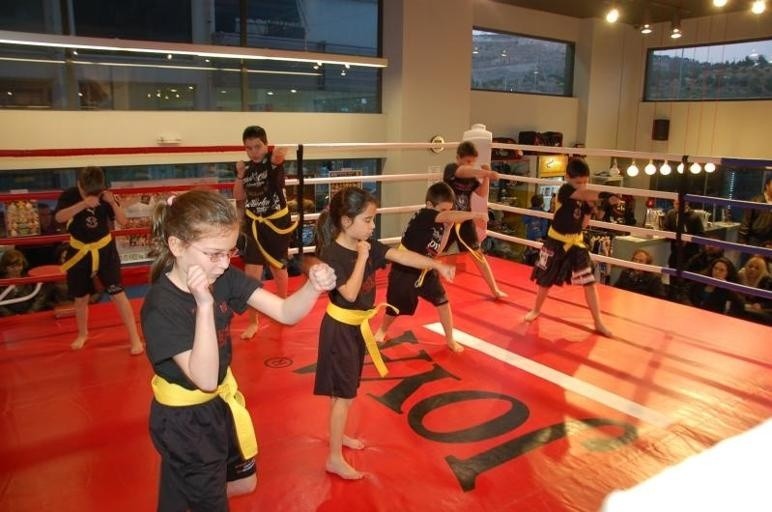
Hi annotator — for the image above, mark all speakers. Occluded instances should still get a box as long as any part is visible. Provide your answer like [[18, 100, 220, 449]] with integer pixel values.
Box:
[[652, 119, 669, 140]]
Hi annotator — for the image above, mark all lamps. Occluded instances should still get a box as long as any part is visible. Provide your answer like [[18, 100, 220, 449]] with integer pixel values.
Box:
[[633, 9, 659, 37], [664, 10, 687, 40]]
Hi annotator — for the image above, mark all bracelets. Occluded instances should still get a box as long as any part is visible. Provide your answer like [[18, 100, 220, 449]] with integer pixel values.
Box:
[[236, 175, 245, 181]]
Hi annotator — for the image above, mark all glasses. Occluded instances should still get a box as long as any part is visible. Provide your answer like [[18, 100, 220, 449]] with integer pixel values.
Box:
[[178, 238, 240, 263]]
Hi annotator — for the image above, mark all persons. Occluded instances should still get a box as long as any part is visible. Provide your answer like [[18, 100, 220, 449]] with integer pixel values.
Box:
[[372, 182, 490, 354], [523, 189, 636, 285], [523, 158, 627, 339], [138, 189, 338, 510], [612, 175, 771, 327], [232, 125, 293, 341], [436, 141, 509, 299], [485, 173, 518, 259], [282, 187, 337, 248], [312, 186, 459, 483], [51, 167, 147, 357], [1, 201, 103, 319]]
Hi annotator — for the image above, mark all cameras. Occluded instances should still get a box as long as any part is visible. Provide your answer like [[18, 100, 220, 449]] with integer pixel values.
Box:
[[95, 193, 115, 220]]
